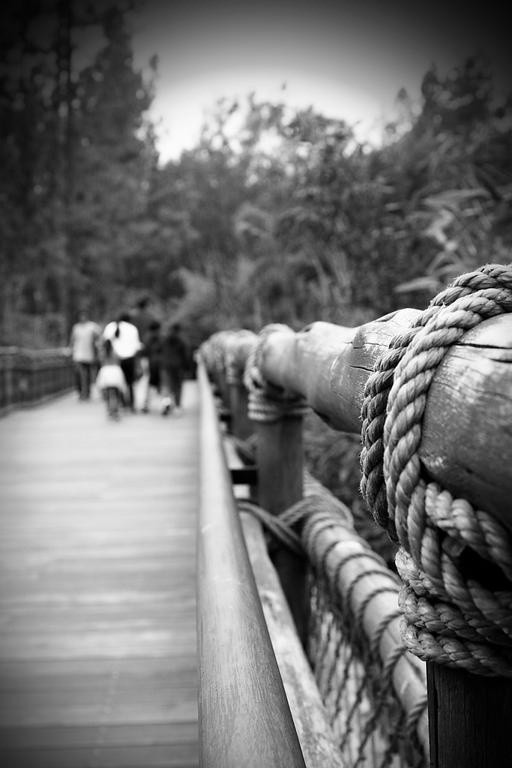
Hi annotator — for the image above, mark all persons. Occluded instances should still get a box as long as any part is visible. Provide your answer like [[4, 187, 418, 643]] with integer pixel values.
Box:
[[63, 298, 188, 419]]
